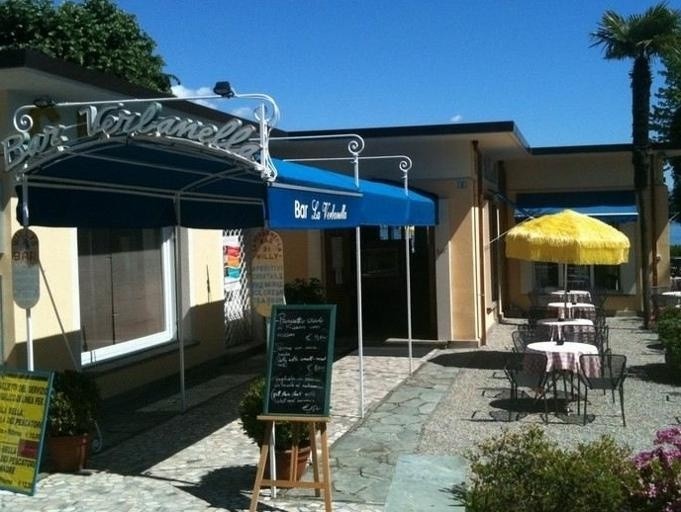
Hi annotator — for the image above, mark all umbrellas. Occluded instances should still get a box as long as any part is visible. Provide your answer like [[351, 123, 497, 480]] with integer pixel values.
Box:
[[505, 207, 632, 320]]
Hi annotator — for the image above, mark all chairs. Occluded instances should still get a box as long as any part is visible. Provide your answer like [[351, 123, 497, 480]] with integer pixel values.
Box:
[[504, 284, 629, 426]]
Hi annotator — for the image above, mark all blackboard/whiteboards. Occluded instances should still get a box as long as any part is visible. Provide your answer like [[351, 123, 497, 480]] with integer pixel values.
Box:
[[263, 305, 337, 416]]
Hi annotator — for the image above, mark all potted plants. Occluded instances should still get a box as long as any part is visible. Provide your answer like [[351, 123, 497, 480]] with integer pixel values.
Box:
[[238, 371, 332, 491], [44, 368, 105, 471]]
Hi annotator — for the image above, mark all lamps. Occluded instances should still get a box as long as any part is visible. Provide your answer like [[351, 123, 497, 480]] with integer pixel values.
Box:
[[213, 81, 235, 98], [33, 95, 56, 109]]
